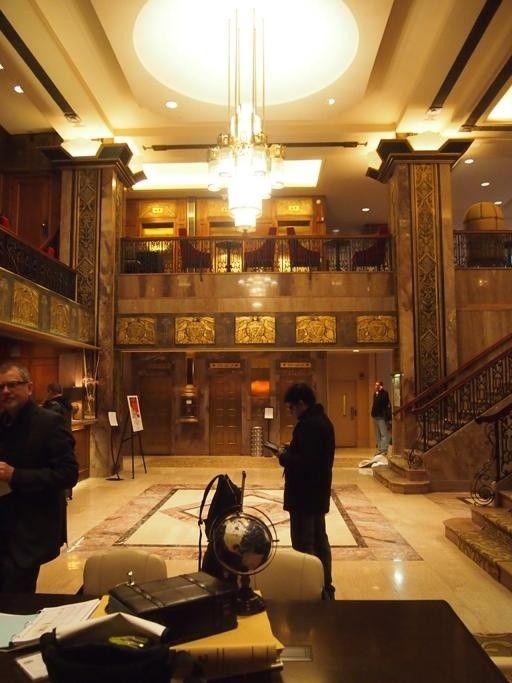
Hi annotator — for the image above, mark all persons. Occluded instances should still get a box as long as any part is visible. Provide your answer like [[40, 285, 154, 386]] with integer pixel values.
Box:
[[41, 381, 73, 501], [0, 364, 82, 594], [369, 381, 391, 454], [264, 383, 335, 597]]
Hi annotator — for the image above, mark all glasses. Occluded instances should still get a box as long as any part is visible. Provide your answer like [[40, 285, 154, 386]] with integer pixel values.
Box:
[[0, 380, 28, 391]]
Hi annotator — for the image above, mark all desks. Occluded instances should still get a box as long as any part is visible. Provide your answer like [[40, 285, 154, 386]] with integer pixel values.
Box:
[[216, 241, 241, 272], [323, 240, 350, 271], [0, 594, 509, 683]]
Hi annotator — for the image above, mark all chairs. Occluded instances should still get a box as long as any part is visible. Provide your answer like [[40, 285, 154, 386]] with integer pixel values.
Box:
[[238, 548, 326, 600], [179, 228, 213, 272], [287, 228, 321, 272], [83, 549, 167, 599], [352, 225, 388, 272], [244, 227, 276, 272]]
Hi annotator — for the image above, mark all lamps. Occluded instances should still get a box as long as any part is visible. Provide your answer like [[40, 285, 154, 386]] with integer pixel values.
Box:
[[206, 7, 287, 234]]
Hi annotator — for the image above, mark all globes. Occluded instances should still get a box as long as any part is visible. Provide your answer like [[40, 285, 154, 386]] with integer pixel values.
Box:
[[207, 504, 280, 616]]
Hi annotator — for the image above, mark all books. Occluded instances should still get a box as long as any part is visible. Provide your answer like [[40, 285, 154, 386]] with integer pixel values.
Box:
[[0, 597, 101, 650], [171, 589, 276, 663], [173, 631, 285, 673]]
